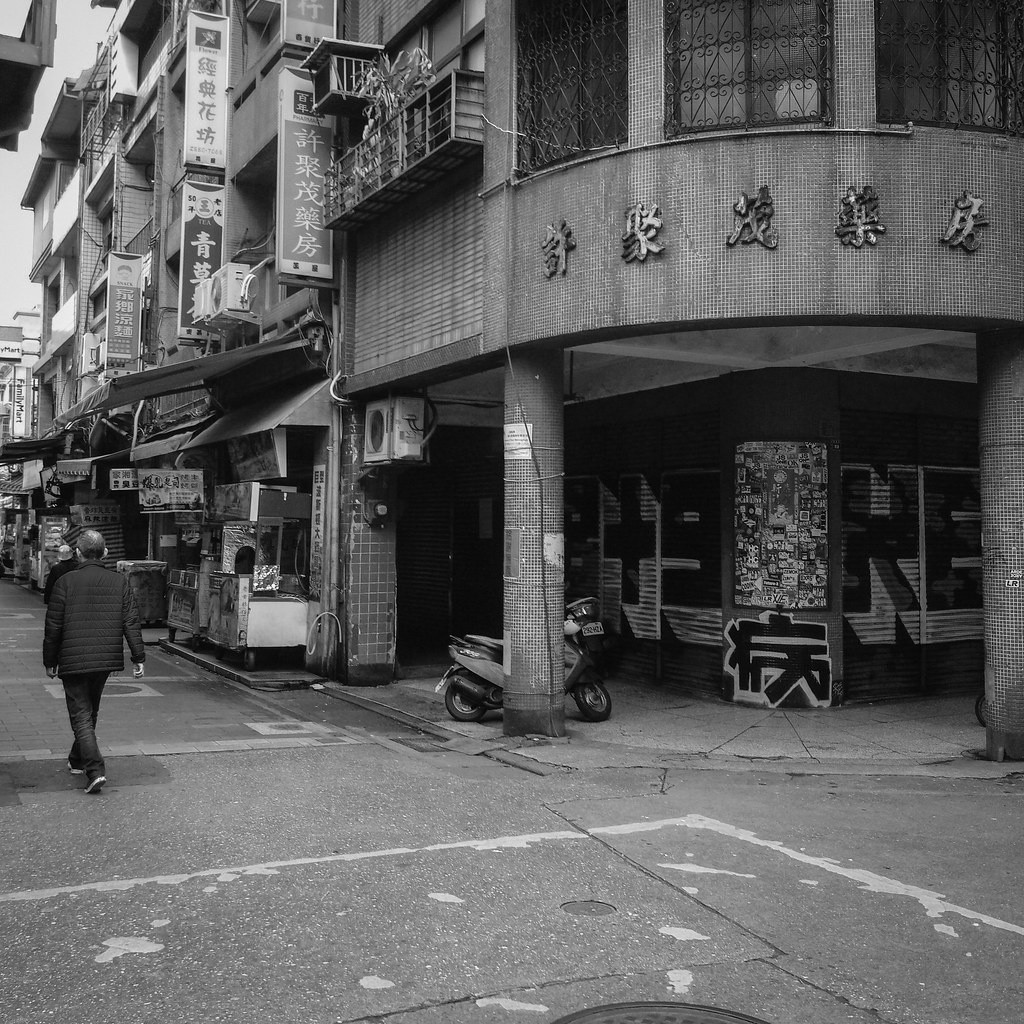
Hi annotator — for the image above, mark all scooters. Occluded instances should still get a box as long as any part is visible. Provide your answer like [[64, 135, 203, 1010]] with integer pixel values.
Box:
[[564, 595, 607, 675], [435, 611, 613, 722]]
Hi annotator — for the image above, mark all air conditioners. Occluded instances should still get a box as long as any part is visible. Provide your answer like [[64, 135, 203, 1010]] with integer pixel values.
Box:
[[310, 54, 378, 120], [209, 262, 250, 314], [362, 396, 425, 464], [95, 342, 107, 369]]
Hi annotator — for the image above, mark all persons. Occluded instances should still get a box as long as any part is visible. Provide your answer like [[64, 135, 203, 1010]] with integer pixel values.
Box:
[[42, 530, 145, 794]]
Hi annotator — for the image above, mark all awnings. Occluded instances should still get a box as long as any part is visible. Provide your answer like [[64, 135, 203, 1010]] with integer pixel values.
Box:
[[0, 334, 333, 495]]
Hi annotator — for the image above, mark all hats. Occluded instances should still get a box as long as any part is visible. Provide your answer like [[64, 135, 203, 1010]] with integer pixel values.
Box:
[[57, 545, 74, 560]]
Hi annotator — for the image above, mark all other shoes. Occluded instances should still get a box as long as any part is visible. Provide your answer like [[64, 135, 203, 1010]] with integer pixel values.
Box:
[[67, 762, 84, 773], [85, 774, 106, 794]]
